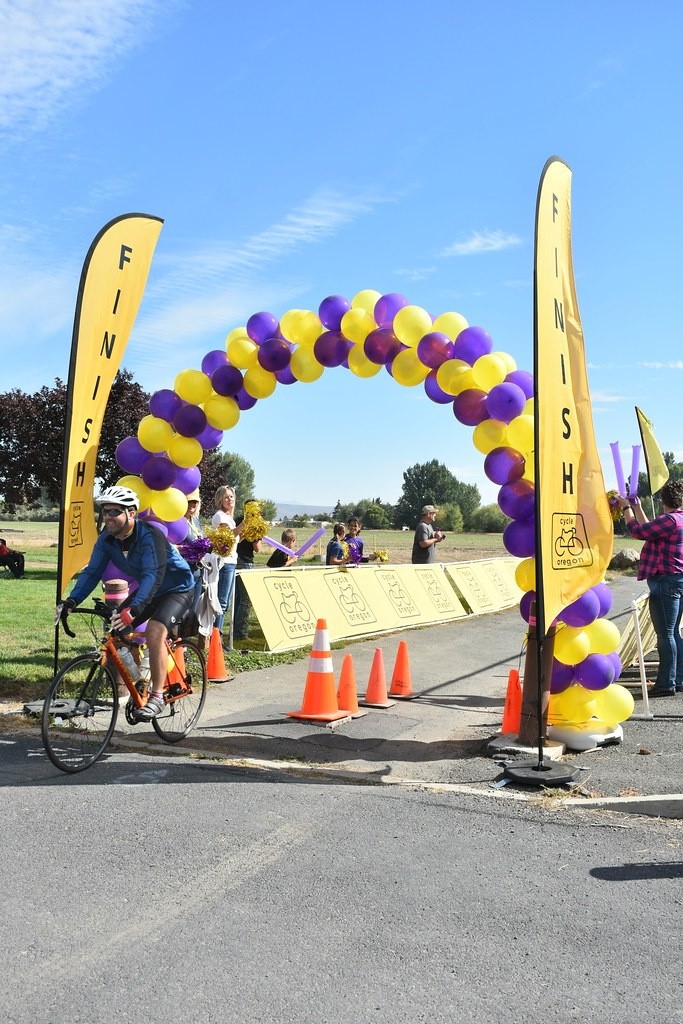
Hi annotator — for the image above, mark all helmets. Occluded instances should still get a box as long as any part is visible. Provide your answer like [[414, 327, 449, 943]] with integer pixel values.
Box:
[[94, 486, 139, 512]]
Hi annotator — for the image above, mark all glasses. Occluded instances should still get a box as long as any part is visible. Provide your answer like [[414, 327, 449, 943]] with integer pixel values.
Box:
[[101, 508, 128, 516]]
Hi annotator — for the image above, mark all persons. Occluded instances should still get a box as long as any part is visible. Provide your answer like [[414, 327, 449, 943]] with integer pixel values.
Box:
[[57, 486, 196, 718], [267, 529, 295, 571], [180, 488, 212, 612], [617, 480, 683, 698], [326, 519, 350, 565], [0, 539, 26, 579], [345, 517, 378, 563], [412, 505, 445, 564], [213, 485, 245, 650], [229, 499, 259, 639]]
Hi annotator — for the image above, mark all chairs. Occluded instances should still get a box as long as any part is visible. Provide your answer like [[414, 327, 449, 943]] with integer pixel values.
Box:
[[0, 551, 26, 578]]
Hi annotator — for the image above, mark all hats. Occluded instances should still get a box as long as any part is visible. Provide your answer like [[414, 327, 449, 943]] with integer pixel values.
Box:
[[421, 504, 439, 515], [186, 487, 201, 502]]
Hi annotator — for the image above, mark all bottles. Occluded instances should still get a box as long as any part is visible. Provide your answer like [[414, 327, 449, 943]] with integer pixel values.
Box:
[[117, 645, 141, 681], [140, 658, 151, 680]]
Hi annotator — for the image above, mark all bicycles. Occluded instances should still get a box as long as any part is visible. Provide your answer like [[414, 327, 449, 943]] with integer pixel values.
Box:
[[42, 599, 208, 773]]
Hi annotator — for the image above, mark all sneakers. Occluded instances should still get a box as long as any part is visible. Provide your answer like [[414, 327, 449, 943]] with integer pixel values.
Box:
[[132, 697, 167, 718]]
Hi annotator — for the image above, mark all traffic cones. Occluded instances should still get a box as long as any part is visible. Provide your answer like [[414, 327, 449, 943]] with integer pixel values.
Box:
[[491, 669, 524, 737], [358, 647, 396, 709], [336, 652, 369, 719], [387, 640, 421, 700], [202, 626, 236, 683], [164, 637, 190, 694], [277, 618, 351, 721]]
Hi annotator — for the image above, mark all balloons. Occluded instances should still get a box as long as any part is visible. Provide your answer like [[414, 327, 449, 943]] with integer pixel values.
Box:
[[610, 441, 627, 499], [629, 445, 641, 499], [114, 289, 635, 726]]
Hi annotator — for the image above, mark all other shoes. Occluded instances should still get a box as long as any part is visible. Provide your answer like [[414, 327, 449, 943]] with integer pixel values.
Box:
[[233, 635, 259, 644], [677, 683, 683, 693], [648, 686, 675, 697]]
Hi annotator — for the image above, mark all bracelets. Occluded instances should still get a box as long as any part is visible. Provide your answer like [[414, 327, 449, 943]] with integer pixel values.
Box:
[[623, 505, 631, 511]]
